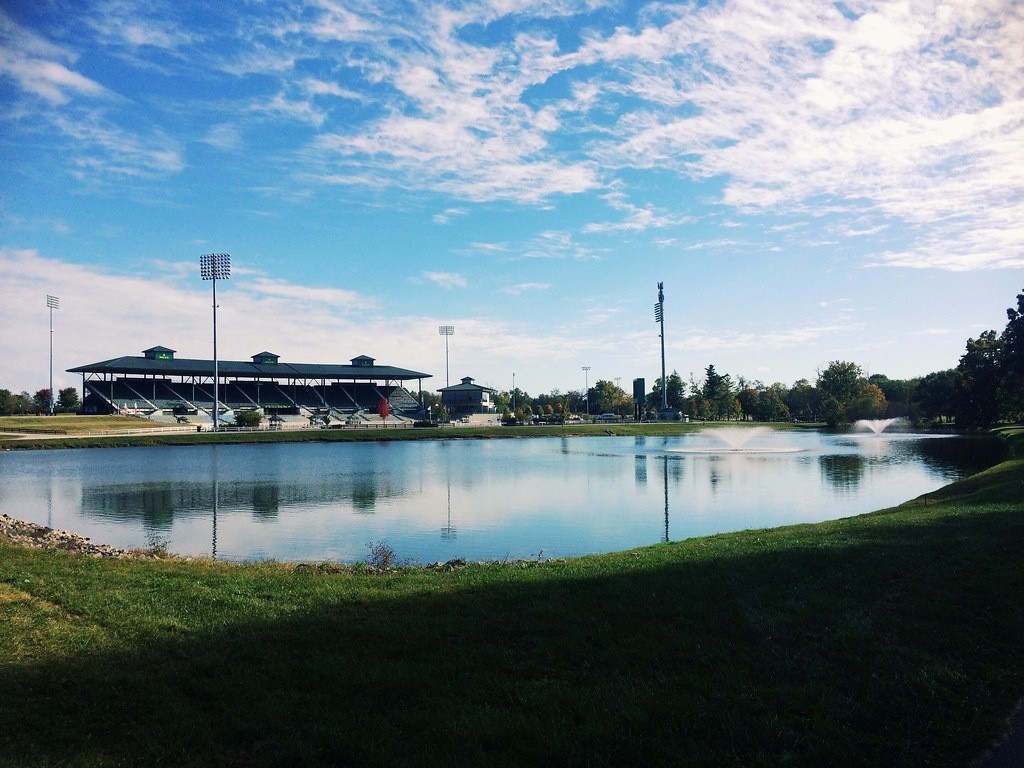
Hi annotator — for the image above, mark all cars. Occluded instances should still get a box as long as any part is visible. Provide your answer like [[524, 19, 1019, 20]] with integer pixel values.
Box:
[[600, 413, 615, 420], [616, 412, 713, 423]]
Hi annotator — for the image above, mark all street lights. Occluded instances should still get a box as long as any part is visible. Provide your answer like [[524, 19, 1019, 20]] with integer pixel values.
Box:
[[614, 376, 622, 414], [512, 372, 516, 412], [198, 251, 232, 426], [439, 325, 455, 388], [654, 281, 668, 409], [46, 292, 60, 416], [581, 365, 592, 415]]
